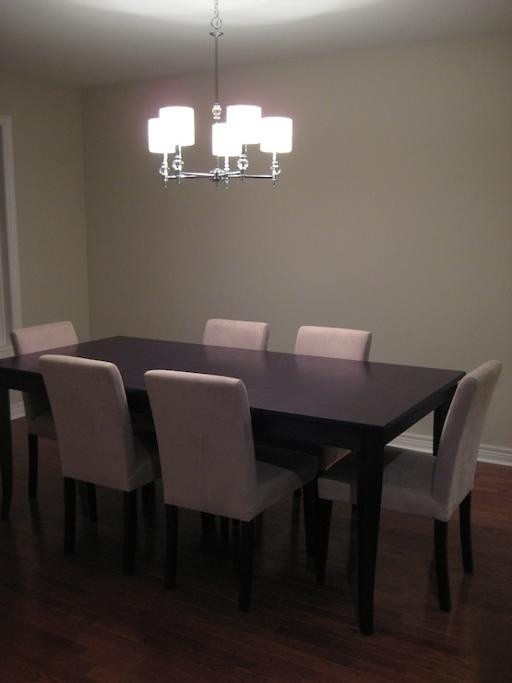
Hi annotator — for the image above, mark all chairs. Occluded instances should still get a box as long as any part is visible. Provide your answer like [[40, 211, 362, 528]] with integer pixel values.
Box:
[[203, 318, 271, 350], [145, 370, 319, 611], [38, 354, 211, 574], [315, 361, 502, 609], [259, 325, 372, 573], [9, 320, 97, 500]]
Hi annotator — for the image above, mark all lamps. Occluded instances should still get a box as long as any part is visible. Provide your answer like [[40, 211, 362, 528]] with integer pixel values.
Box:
[[147, 0, 293, 190]]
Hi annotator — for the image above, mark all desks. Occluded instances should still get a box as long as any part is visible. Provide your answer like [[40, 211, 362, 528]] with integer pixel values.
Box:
[[0, 333, 474, 635]]
